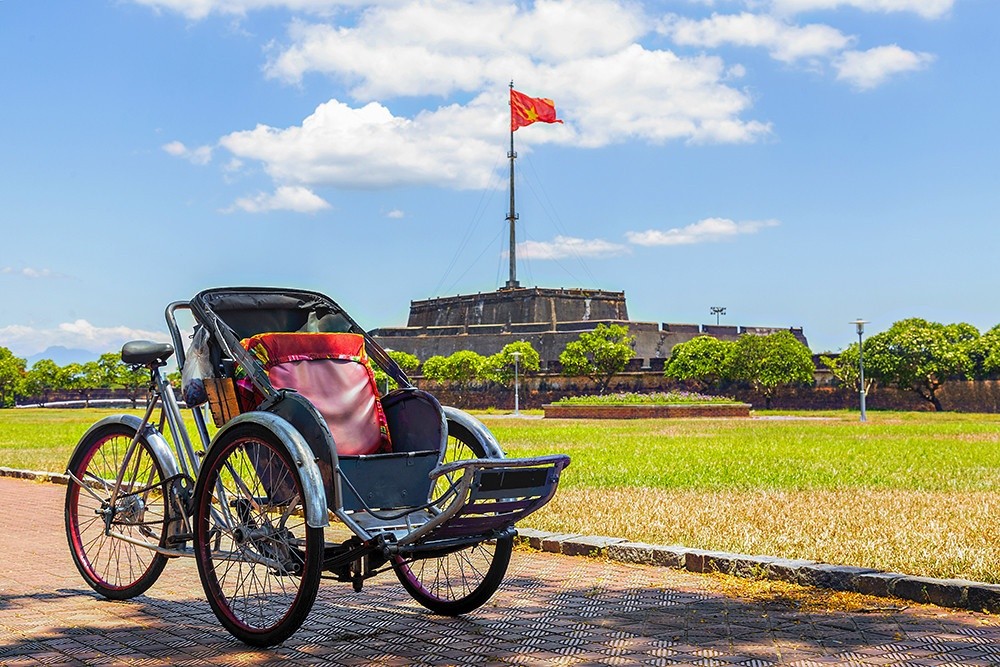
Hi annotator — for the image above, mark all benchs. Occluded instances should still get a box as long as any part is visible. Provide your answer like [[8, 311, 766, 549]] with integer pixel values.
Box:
[[232, 329, 451, 511]]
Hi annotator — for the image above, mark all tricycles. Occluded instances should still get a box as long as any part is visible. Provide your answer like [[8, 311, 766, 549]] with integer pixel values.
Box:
[[63, 286, 572, 648]]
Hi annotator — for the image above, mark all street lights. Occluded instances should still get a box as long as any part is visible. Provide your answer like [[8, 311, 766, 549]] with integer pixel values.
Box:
[[509, 350, 523, 416], [848, 318, 871, 421]]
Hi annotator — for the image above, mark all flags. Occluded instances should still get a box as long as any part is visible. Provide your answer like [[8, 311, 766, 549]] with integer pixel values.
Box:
[[512, 89, 563, 131]]
